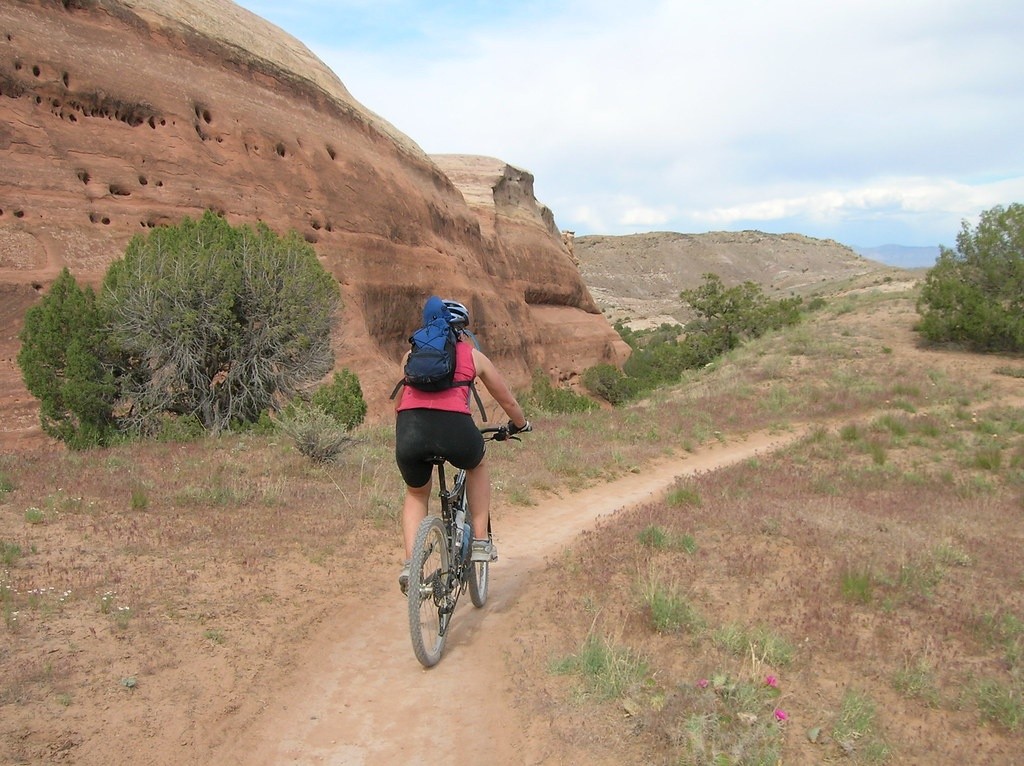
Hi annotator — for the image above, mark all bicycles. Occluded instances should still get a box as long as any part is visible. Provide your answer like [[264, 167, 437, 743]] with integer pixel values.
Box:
[[402, 424, 535, 668]]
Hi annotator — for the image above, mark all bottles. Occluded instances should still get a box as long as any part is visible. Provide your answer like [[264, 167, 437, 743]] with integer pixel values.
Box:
[[462, 522, 470, 560], [455, 505, 465, 549]]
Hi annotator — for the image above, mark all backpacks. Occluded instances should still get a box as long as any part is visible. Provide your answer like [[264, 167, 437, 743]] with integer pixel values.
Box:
[[403, 295, 456, 392]]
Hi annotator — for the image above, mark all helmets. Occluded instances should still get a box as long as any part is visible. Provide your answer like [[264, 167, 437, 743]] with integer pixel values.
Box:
[[441, 299, 470, 327]]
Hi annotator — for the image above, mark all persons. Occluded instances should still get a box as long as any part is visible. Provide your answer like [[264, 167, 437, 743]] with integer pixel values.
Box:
[[394, 300, 531, 596]]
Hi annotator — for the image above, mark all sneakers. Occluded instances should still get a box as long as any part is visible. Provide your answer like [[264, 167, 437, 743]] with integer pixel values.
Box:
[[470, 535, 498, 563], [399, 562, 426, 595]]
